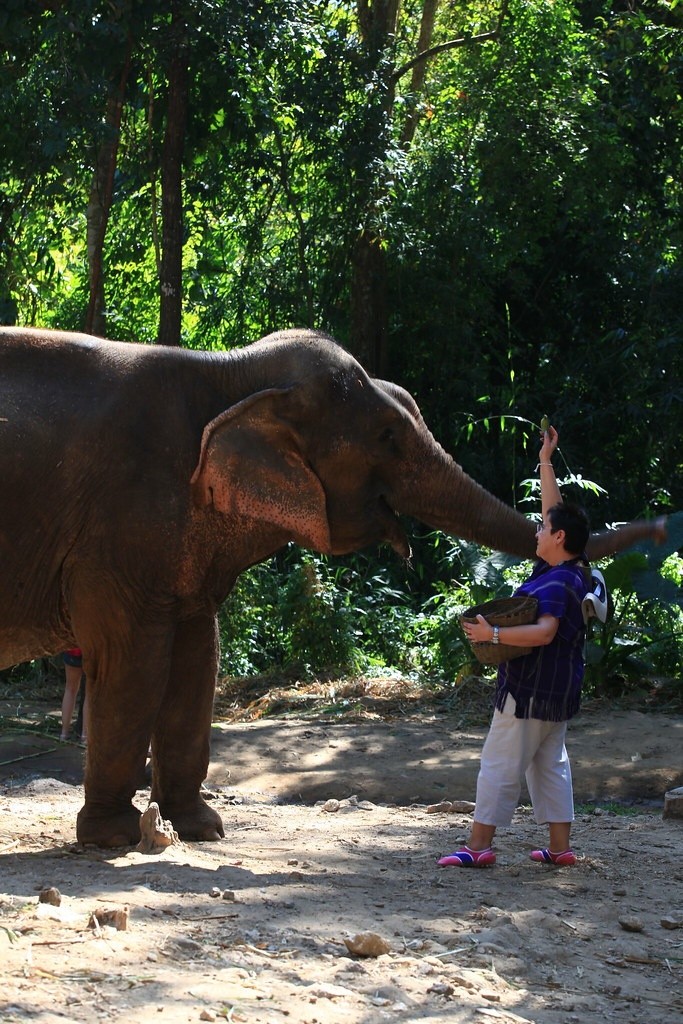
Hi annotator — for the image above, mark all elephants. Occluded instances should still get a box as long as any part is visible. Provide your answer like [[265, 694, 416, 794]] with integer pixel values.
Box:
[[0, 328, 666, 847]]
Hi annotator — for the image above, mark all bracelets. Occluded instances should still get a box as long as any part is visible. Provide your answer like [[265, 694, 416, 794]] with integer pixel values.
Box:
[[534, 462, 553, 472], [492, 626, 499, 644]]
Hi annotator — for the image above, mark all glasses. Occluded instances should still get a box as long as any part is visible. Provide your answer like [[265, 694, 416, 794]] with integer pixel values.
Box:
[[537, 524, 557, 533]]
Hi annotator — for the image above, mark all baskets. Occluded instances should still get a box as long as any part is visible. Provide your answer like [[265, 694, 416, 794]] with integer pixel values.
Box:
[[461, 596, 537, 666]]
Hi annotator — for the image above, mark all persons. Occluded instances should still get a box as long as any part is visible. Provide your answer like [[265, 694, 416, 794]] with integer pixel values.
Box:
[[60, 648, 90, 743], [436, 426, 591, 866]]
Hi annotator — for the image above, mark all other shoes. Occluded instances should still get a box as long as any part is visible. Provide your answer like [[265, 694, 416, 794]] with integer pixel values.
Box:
[[437, 845, 496, 867], [530, 849, 576, 865], [60, 734, 69, 743], [80, 735, 87, 746]]
